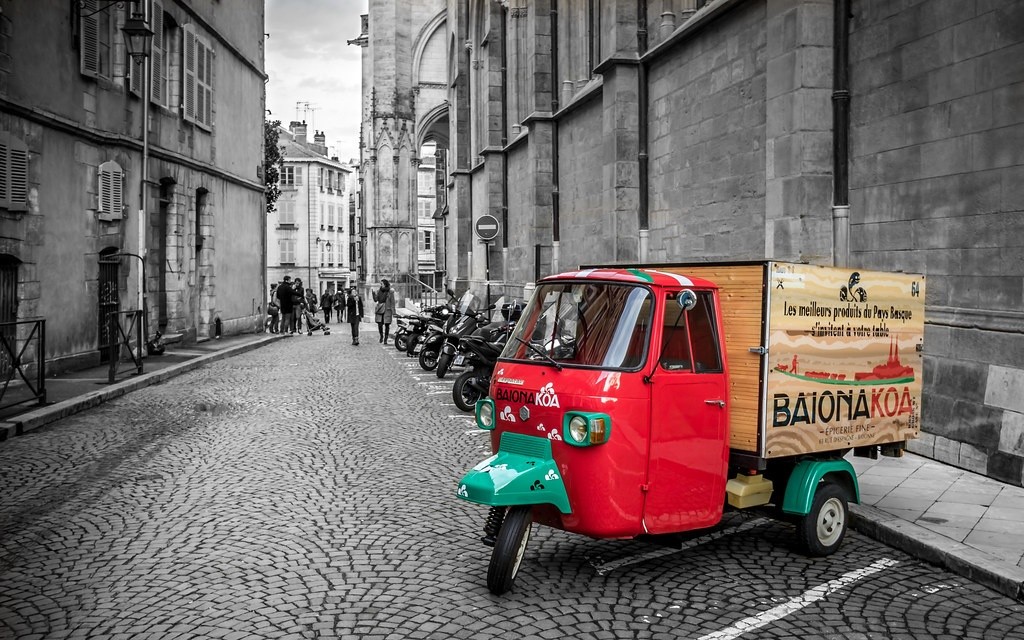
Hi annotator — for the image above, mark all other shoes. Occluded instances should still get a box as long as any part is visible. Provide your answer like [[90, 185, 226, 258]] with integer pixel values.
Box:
[[384, 338, 387, 343], [323, 328, 330, 331], [290, 331, 293, 334], [270, 330, 274, 333], [380, 335, 383, 343], [285, 330, 288, 332], [281, 330, 283, 333], [276, 331, 279, 334], [352, 338, 359, 345], [298, 329, 303, 334]]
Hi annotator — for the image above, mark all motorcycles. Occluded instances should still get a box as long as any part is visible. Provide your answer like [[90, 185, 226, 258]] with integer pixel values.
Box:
[[391, 288, 577, 412]]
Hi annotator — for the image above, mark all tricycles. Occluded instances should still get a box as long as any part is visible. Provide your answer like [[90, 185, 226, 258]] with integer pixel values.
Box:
[[455, 258, 928, 596]]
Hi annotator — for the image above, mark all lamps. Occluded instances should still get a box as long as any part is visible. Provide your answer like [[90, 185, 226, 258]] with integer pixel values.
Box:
[[70, 0, 154, 66]]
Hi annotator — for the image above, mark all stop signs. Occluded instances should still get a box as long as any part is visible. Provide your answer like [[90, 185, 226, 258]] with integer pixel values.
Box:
[[474, 215, 500, 241]]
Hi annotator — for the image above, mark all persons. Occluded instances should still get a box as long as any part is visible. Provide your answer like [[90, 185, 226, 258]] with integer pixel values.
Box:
[[269, 276, 397, 345]]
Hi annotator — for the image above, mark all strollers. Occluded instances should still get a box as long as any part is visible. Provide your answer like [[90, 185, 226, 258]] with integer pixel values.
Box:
[[297, 305, 331, 336]]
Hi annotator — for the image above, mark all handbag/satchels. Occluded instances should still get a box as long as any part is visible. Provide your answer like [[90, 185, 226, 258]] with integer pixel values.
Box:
[[334, 300, 338, 307], [267, 305, 278, 315], [375, 303, 385, 314]]
